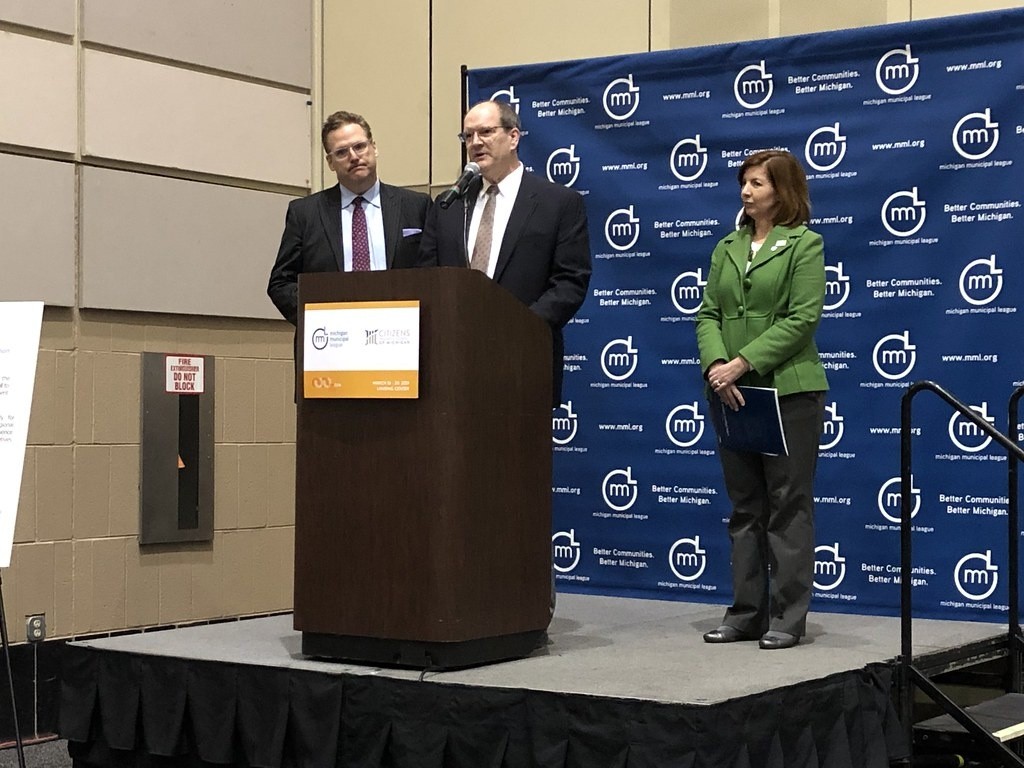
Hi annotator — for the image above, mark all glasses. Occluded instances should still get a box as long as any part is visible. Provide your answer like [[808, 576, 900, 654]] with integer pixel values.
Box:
[[328, 138, 373, 159], [458, 126, 513, 142]]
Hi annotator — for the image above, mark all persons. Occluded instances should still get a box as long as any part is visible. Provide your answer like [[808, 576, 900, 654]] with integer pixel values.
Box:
[[413, 100, 594, 409], [266, 110, 433, 405], [694, 149, 831, 649]]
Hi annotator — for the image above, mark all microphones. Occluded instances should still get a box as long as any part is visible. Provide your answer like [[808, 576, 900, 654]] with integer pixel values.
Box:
[[439, 161, 480, 210]]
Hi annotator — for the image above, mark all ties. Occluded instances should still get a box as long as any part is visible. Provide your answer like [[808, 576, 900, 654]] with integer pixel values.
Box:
[[351, 196, 370, 271], [470, 184, 500, 275]]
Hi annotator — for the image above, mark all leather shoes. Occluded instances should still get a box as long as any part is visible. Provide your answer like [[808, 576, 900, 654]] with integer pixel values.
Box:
[[703, 629, 764, 643], [759, 633, 801, 649]]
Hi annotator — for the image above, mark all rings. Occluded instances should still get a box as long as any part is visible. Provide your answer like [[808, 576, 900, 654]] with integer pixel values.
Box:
[[716, 379, 721, 386]]
